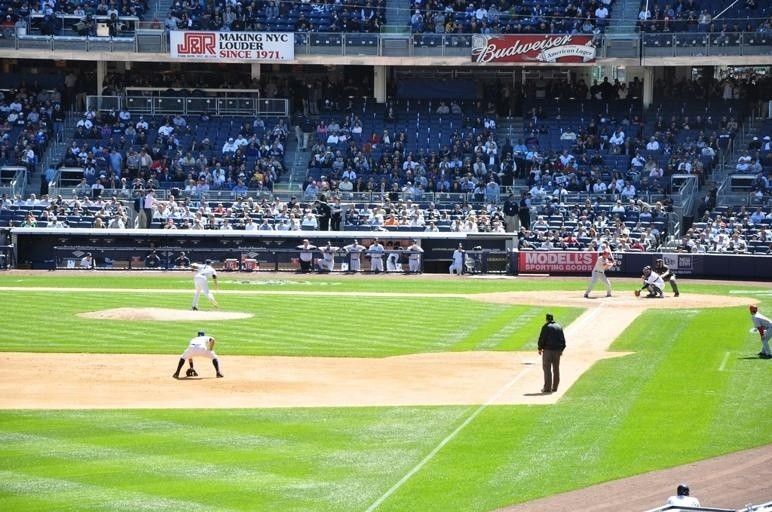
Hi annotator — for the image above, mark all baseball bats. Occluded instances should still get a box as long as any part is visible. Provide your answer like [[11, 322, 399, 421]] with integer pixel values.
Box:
[[608, 245, 615, 263]]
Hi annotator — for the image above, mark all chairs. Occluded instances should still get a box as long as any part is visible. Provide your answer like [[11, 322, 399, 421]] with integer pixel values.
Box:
[[0, 0, 772, 268]]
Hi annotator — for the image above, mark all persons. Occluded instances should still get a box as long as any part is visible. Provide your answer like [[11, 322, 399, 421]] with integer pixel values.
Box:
[[172, 330, 224, 379], [664, 483, 701, 507], [634, 258, 680, 298], [749, 303, 772, 359], [191, 259, 219, 310], [0, 1, 770, 44], [1, 58, 772, 276], [583, 250, 617, 298], [537, 313, 566, 393]]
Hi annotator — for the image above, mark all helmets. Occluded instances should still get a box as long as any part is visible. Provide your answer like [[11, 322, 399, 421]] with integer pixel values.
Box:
[[750, 305, 757, 312], [604, 249, 611, 256], [676, 484, 689, 496], [643, 259, 665, 275], [457, 243, 463, 248], [326, 238, 418, 251]]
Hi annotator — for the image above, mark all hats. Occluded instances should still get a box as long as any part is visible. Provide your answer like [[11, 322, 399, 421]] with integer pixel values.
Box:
[[197, 330, 205, 336], [546, 314, 553, 321], [205, 259, 211, 264]]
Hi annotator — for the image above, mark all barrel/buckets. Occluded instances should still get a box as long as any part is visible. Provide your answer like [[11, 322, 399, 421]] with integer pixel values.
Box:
[[226, 258, 237, 270], [291, 257, 299, 267], [245, 258, 257, 269]]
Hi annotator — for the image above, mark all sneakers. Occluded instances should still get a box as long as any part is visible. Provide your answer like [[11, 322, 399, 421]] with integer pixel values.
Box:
[[216, 373, 224, 378], [541, 389, 557, 392], [758, 352, 771, 359], [607, 294, 612, 297], [192, 307, 198, 311], [584, 294, 588, 298], [674, 293, 680, 297], [647, 293, 664, 298], [173, 373, 180, 379]]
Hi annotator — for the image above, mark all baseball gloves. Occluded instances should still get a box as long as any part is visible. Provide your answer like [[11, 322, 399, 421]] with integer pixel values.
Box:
[[635, 290, 639, 295]]
